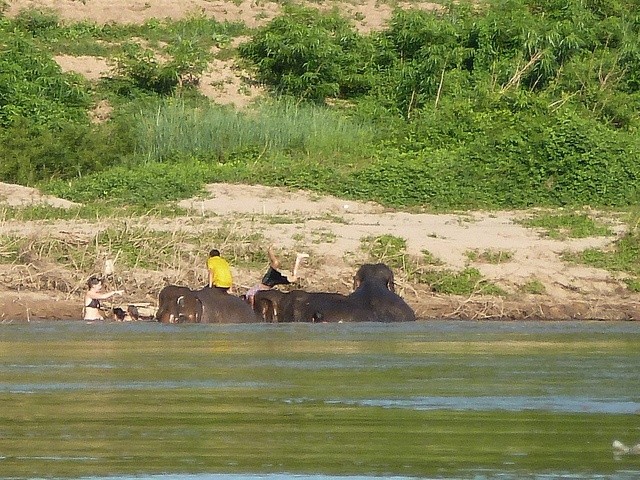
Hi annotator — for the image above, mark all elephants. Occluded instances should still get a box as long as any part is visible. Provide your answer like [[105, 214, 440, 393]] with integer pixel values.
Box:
[[313, 263, 415, 323], [156, 284, 257, 324], [277, 290, 347, 323], [253, 289, 284, 323]]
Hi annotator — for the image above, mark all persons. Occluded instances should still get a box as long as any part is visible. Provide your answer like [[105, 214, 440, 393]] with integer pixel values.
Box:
[[112, 308, 126, 321], [238, 243, 309, 312], [84, 278, 125, 321], [204, 249, 234, 294], [124, 305, 142, 321]]
